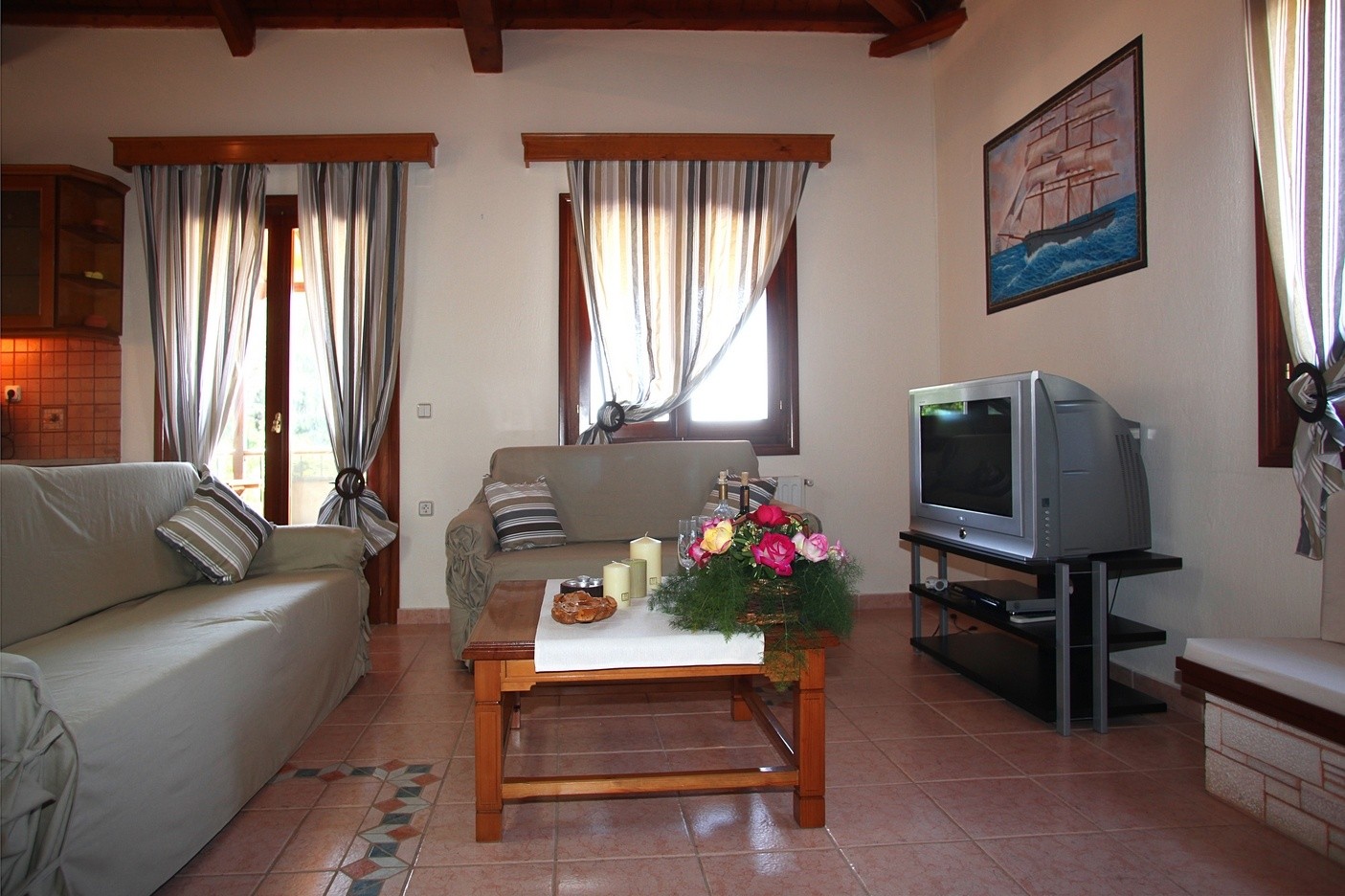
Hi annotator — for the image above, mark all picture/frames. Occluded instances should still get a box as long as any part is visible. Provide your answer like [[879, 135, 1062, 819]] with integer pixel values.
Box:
[[984, 34, 1149, 316]]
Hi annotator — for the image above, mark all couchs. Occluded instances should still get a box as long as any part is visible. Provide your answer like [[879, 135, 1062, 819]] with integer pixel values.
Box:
[[0, 459, 370, 895], [445, 439, 762, 658]]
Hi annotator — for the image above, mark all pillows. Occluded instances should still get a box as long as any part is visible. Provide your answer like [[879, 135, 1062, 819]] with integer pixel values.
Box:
[[480, 470, 568, 553], [701, 469, 781, 524], [155, 465, 276, 589]]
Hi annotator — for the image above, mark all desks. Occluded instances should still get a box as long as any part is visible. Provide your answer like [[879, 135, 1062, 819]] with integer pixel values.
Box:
[[461, 575, 840, 843]]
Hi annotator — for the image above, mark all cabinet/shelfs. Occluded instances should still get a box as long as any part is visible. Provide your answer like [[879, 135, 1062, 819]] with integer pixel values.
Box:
[[897, 528, 1184, 736]]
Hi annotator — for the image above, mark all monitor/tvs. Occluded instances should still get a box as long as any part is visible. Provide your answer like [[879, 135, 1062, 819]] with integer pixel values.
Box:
[[909, 369, 1152, 563]]
